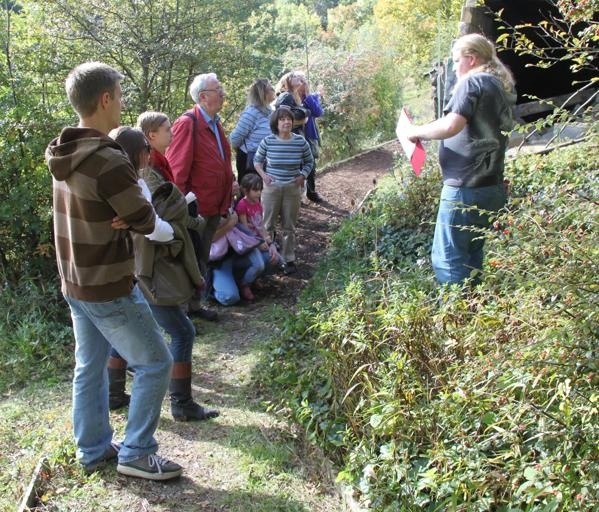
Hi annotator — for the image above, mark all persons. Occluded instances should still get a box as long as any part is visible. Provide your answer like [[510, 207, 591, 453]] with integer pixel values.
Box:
[[165, 71, 323, 319], [43, 61, 186, 480], [407, 32, 517, 305], [107, 126, 220, 424], [137, 111, 173, 184]]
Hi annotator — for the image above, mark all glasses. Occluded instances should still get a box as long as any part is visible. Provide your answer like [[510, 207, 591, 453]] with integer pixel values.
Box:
[[199, 87, 222, 94]]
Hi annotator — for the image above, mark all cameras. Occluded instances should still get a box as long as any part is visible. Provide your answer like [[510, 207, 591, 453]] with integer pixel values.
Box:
[[423, 69, 436, 78]]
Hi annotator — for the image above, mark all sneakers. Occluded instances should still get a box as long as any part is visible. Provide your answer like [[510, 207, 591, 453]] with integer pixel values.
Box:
[[116, 448, 185, 482], [80, 446, 118, 473], [225, 253, 302, 305], [171, 396, 222, 424], [107, 391, 133, 411]]
